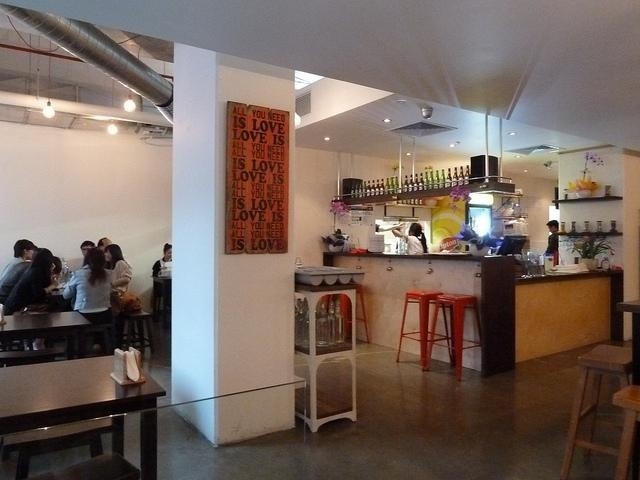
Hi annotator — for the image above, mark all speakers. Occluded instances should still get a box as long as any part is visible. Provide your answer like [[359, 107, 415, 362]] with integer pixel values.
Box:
[[471, 155, 498, 184], [343, 178, 363, 199]]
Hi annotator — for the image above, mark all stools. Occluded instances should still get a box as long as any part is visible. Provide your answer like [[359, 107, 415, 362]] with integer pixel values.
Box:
[[613, 384, 640, 480], [78, 323, 114, 359], [396, 290, 452, 372], [560, 343, 633, 480], [323, 283, 370, 345], [425, 292, 482, 382]]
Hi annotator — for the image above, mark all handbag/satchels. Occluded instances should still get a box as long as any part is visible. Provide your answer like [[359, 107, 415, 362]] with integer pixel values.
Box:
[[111, 288, 123, 318], [123, 290, 142, 317]]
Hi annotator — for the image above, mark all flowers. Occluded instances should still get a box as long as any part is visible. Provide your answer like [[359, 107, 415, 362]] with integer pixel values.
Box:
[[568, 151, 605, 192], [449, 186, 472, 211], [329, 201, 350, 216], [568, 236, 615, 259], [456, 224, 503, 247]]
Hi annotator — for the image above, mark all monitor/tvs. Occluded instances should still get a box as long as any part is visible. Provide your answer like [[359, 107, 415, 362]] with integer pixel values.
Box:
[[497, 236, 525, 254]]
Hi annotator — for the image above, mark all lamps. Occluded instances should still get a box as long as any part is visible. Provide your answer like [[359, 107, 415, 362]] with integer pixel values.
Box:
[[107, 79, 118, 135], [124, 90, 136, 113], [42, 41, 56, 119]]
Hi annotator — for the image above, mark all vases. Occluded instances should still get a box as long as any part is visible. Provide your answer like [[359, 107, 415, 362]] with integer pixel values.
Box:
[[581, 258, 599, 271], [470, 245, 490, 257], [576, 189, 592, 199]]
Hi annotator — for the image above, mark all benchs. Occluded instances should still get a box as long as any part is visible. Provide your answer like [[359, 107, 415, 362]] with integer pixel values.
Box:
[[28, 453, 141, 480], [2, 418, 113, 480], [122, 312, 155, 355], [1, 346, 67, 367]]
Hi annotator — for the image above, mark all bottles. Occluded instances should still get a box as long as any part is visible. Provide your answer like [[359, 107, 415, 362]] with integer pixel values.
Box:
[[408, 174, 414, 192], [434, 170, 439, 189], [413, 173, 418, 191], [424, 171, 428, 190], [371, 180, 375, 196], [418, 173, 424, 191], [355, 184, 358, 198], [351, 185, 354, 198], [390, 177, 395, 194], [296, 297, 346, 350], [571, 221, 576, 233], [358, 183, 363, 198], [439, 169, 444, 188], [363, 181, 367, 197], [429, 170, 433, 190], [445, 168, 452, 188], [584, 220, 589, 232], [561, 222, 565, 233], [366, 180, 371, 196], [375, 179, 379, 196], [379, 179, 384, 195], [384, 177, 389, 194], [604, 184, 611, 196], [394, 176, 399, 194], [596, 220, 602, 232], [464, 165, 470, 185], [453, 166, 458, 187], [458, 166, 464, 186], [563, 189, 569, 199], [404, 175, 408, 193], [610, 220, 616, 232]]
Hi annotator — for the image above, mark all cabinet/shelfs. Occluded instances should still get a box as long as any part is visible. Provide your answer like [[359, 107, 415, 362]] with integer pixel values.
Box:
[[552, 195, 623, 237], [294, 281, 359, 433]]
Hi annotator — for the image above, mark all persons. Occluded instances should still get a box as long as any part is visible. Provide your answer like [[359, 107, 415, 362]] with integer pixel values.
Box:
[[1, 247, 68, 351], [63, 248, 117, 354], [81, 241, 96, 257], [392, 221, 428, 254], [104, 244, 133, 351], [542, 219, 559, 267], [98, 237, 113, 253], [373, 222, 405, 233], [1, 256, 62, 307], [1, 237, 37, 280], [152, 242, 172, 276]]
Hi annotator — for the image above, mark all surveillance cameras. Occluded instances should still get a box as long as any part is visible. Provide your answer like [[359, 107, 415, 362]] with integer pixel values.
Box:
[[421, 108, 433, 119]]
